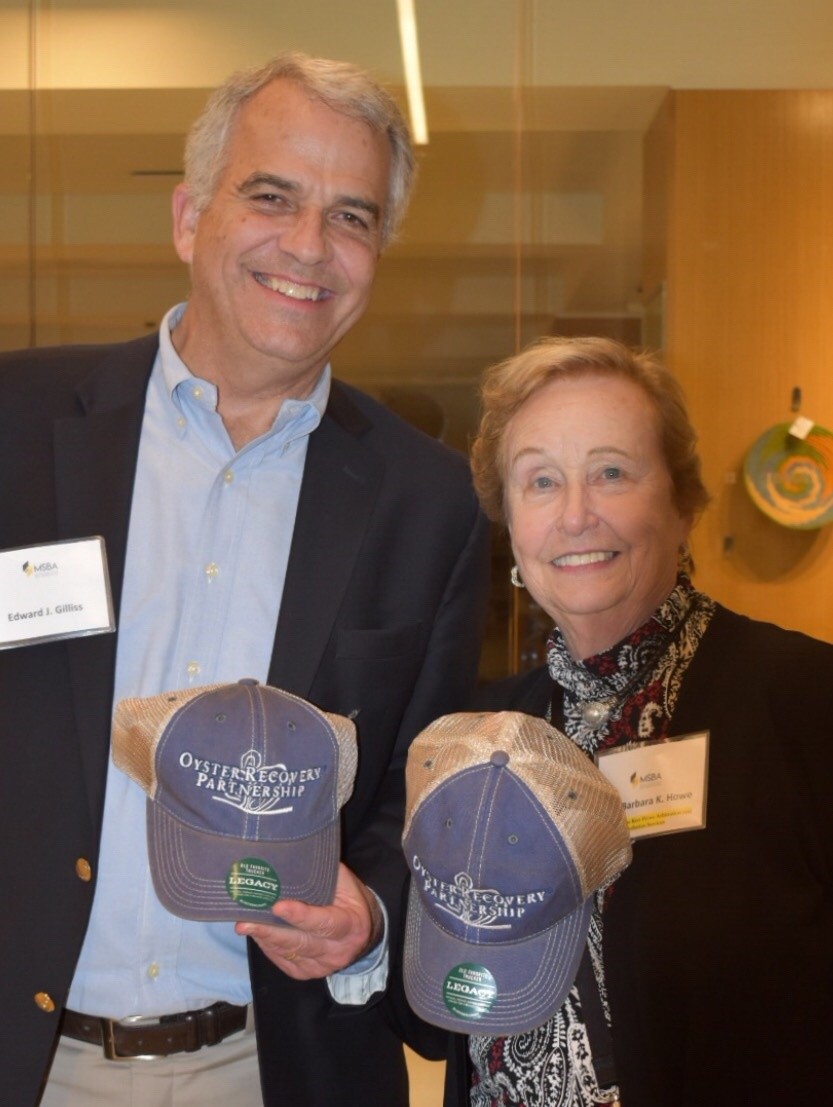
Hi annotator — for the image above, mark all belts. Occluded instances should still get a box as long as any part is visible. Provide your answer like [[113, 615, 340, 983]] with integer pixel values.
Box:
[[59, 1001, 247, 1062]]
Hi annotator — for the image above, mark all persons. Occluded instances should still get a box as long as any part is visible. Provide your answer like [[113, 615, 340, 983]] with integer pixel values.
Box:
[[0, 52, 489, 1107], [427, 338, 833, 1107]]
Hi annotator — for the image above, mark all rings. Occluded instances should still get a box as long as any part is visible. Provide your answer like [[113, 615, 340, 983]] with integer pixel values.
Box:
[[290, 954, 296, 960]]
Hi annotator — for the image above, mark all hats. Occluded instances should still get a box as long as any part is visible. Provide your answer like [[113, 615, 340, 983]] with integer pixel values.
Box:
[[112, 678, 358, 928], [401, 710, 633, 1037]]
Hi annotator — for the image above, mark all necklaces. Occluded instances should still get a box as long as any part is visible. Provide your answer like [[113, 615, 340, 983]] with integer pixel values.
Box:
[[581, 596, 697, 728]]
